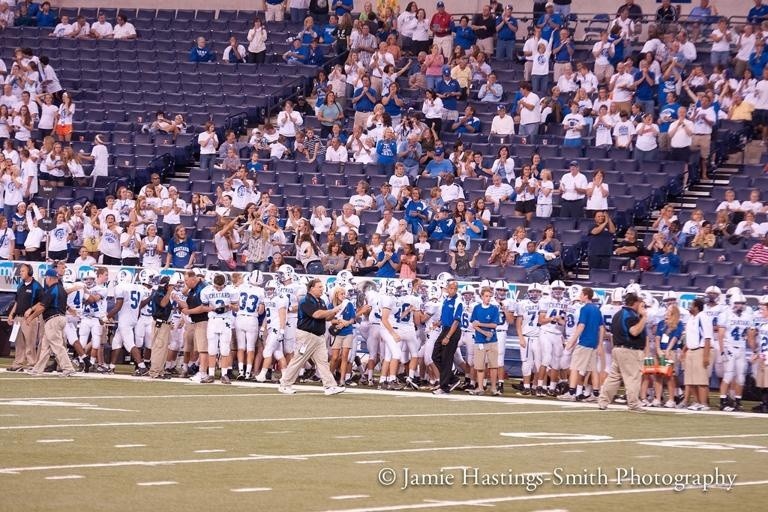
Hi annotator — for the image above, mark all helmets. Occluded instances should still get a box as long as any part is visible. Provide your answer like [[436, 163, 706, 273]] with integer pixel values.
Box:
[[152, 271, 159, 283], [172, 273, 183, 286], [527, 280, 678, 303], [139, 269, 153, 284], [82, 270, 97, 283], [386, 272, 508, 300], [117, 270, 130, 284], [192, 268, 205, 277], [705, 287, 767, 311]]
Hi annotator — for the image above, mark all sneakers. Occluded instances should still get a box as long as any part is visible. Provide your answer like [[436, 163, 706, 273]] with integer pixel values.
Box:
[[132, 361, 271, 384], [720, 404, 735, 411], [299, 372, 321, 383], [7, 357, 115, 377], [339, 373, 504, 395], [732, 402, 743, 410], [325, 386, 346, 396], [511, 381, 599, 402], [279, 387, 296, 394], [615, 394, 711, 414], [752, 403, 768, 413]]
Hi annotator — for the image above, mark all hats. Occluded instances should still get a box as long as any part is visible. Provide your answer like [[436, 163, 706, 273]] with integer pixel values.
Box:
[[41, 269, 58, 277], [497, 104, 507, 111], [436, 1, 444, 7], [465, 207, 476, 214], [545, 1, 554, 9], [505, 5, 512, 11], [435, 147, 444, 154], [442, 67, 450, 76], [570, 160, 578, 167], [248, 269, 264, 286], [278, 264, 295, 283], [336, 269, 353, 292], [440, 205, 450, 212]]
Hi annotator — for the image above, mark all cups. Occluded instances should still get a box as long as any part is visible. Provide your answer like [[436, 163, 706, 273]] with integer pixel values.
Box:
[[137, 116, 142, 122], [312, 176, 318, 184], [336, 179, 341, 186], [500, 136, 548, 146], [80, 149, 84, 153], [492, 222, 497, 227], [79, 136, 85, 141], [435, 256, 440, 263], [164, 139, 168, 144], [612, 251, 704, 283], [263, 164, 267, 171], [121, 138, 126, 143], [125, 160, 130, 166], [269, 188, 273, 194]]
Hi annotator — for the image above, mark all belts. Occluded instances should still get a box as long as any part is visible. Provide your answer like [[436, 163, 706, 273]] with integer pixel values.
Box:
[[42, 314, 58, 323], [610, 343, 643, 353]]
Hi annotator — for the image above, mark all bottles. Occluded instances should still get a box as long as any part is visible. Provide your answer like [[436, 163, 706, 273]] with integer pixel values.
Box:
[[648, 357, 653, 365], [656, 353, 664, 367], [485, 329, 494, 342], [665, 360, 674, 366], [644, 357, 649, 366]]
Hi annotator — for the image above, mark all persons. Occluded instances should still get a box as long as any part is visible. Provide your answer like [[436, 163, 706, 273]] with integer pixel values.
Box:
[[1, 0, 768, 417]]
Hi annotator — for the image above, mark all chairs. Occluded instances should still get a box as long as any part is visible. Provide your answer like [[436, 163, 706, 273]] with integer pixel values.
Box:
[[0, 0, 768, 296]]
